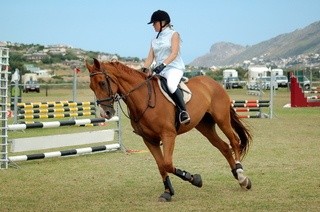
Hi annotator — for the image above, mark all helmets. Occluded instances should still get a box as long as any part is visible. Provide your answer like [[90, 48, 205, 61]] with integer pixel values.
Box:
[[147, 10, 171, 24]]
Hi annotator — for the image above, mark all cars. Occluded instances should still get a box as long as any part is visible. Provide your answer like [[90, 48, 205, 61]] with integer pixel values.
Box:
[[23, 80, 40, 93], [247, 82, 259, 90], [296, 76, 311, 90], [275, 76, 288, 87], [257, 77, 278, 90], [226, 77, 242, 88]]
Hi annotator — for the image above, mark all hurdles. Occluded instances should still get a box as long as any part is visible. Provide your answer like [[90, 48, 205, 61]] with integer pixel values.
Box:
[[0, 49, 320, 169]]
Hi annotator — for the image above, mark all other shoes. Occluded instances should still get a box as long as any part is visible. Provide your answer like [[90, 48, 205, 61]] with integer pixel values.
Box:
[[179, 111, 190, 125]]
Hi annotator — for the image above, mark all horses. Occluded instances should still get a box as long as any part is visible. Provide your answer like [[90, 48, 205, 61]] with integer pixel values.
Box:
[[86, 58, 253, 202]]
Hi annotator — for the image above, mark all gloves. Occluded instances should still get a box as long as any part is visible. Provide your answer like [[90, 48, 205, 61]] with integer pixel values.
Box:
[[152, 63, 166, 74], [140, 67, 147, 73]]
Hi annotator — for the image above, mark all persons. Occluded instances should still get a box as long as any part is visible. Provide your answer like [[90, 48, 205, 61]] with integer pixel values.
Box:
[[141, 10, 190, 126]]
